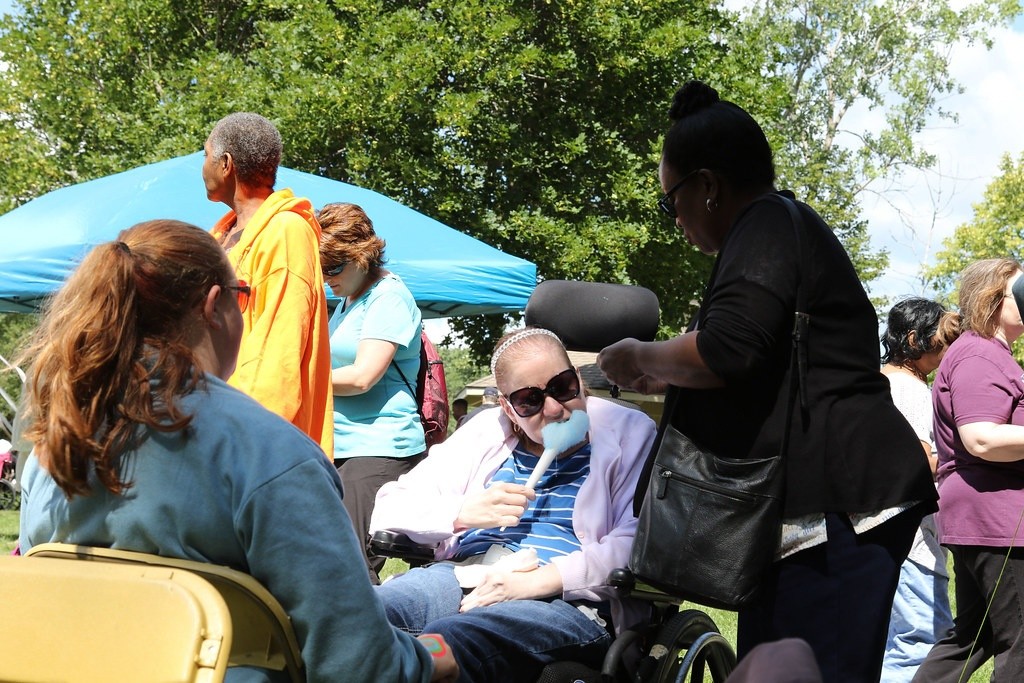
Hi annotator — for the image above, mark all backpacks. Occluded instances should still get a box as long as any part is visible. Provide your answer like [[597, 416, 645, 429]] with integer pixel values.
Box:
[[392, 330, 450, 454]]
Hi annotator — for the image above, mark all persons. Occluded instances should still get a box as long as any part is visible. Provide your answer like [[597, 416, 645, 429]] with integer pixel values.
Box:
[[316, 202, 448, 586], [595, 82, 936, 683], [881, 297, 954, 683], [452, 387, 499, 431], [18, 219, 457, 683], [200, 111, 335, 466], [910, 258, 1024, 683], [371, 327, 657, 683]]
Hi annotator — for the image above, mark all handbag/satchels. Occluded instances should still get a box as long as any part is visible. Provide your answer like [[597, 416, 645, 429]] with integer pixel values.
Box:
[[632, 423, 783, 606]]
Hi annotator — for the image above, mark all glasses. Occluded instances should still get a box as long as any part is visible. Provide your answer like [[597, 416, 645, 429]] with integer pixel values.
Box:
[[223, 285, 250, 312], [504, 365, 580, 417], [321, 261, 350, 276]]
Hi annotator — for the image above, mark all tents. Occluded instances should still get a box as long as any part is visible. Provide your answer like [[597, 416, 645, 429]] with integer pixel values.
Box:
[[0, 144, 537, 305]]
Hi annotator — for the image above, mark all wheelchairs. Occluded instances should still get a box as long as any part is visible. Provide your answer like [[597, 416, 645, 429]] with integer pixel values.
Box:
[[0, 451, 21, 512], [367, 279, 739, 683]]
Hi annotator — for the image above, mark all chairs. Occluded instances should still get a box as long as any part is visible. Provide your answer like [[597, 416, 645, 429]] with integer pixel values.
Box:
[[0, 542, 305, 683]]
